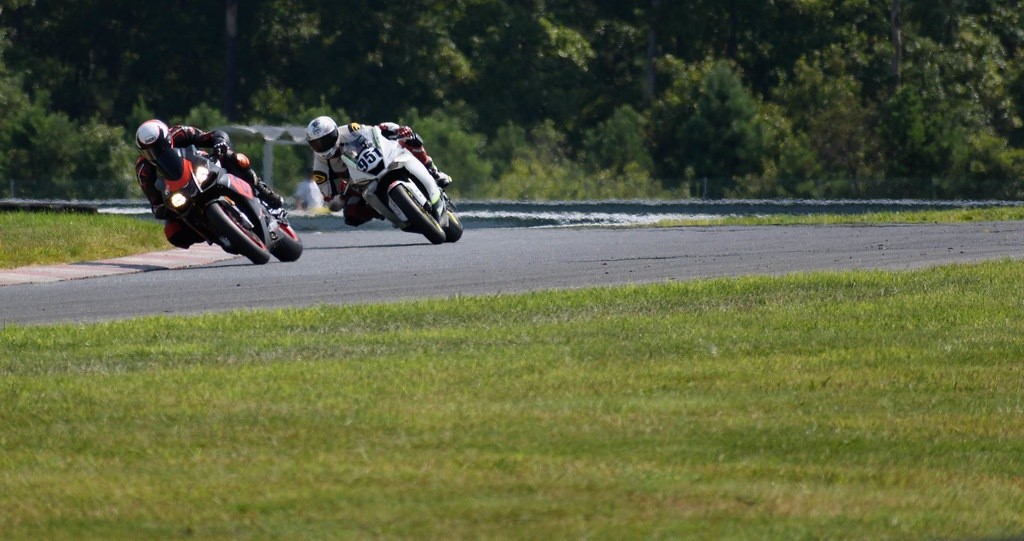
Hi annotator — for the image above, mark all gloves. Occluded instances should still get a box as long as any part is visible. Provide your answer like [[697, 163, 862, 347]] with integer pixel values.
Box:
[[397, 124, 415, 140], [153, 205, 171, 220], [329, 194, 346, 212], [215, 142, 232, 159]]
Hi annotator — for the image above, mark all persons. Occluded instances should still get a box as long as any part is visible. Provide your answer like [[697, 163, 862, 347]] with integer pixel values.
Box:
[[135, 119, 285, 255], [305, 116, 453, 228]]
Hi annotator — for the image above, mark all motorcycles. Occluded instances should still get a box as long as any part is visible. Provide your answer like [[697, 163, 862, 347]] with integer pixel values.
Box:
[[337, 124, 465, 245], [154, 143, 303, 265]]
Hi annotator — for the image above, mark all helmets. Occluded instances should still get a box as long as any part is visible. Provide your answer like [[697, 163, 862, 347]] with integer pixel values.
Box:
[[304, 117, 341, 162], [135, 119, 175, 161]]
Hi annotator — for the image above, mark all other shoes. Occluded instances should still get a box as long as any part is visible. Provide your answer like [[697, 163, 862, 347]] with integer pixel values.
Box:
[[258, 181, 285, 209], [424, 158, 452, 187]]
